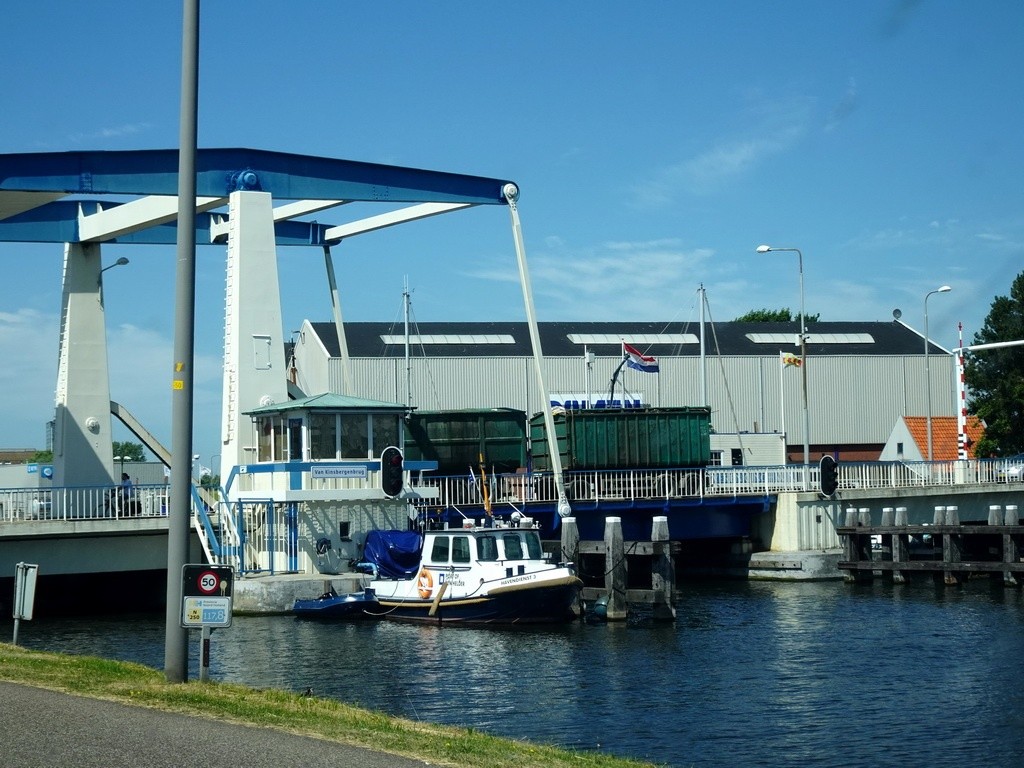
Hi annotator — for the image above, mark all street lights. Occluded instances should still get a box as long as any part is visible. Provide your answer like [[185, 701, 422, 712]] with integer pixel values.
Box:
[[756, 244, 810, 491], [113, 455, 132, 483], [923, 285, 953, 485]]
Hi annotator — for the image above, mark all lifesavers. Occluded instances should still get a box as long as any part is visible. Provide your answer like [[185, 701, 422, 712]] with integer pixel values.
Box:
[[417, 570, 433, 598]]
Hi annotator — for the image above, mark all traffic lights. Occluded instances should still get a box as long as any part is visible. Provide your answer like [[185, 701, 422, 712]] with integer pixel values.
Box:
[[380, 446, 404, 499], [819, 455, 838, 498]]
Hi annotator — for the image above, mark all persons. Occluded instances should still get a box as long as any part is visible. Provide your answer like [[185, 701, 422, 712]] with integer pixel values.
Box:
[[110, 472, 134, 513]]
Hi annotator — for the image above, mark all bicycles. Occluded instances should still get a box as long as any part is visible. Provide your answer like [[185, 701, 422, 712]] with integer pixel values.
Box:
[[94, 488, 141, 518]]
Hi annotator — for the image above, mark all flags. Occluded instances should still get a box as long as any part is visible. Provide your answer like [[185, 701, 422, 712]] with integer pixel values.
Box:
[[624, 342, 659, 374], [782, 353, 802, 368]]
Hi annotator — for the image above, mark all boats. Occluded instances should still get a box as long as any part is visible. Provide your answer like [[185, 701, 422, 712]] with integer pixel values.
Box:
[[370, 453, 584, 625], [293, 584, 381, 622]]
